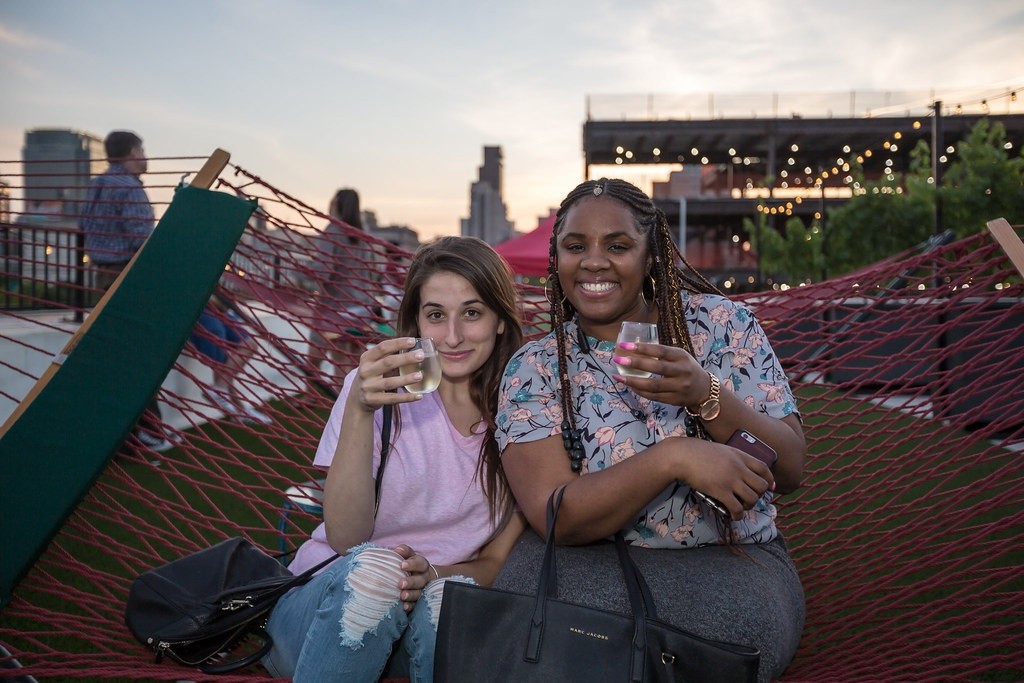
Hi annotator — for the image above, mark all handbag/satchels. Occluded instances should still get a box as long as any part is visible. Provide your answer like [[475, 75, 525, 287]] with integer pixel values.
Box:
[[434, 485, 759, 683], [126, 536, 301, 674]]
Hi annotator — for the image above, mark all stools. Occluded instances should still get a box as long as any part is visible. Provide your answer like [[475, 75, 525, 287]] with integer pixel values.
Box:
[[278, 480, 326, 568]]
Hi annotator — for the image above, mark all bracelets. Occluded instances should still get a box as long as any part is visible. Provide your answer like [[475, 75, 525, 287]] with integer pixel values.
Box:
[[430, 564, 439, 578]]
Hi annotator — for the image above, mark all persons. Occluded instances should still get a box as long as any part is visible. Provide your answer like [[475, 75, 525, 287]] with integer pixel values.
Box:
[[78, 131, 185, 450], [289, 188, 408, 408], [488, 179, 808, 683], [189, 288, 273, 425], [257, 236, 527, 683]]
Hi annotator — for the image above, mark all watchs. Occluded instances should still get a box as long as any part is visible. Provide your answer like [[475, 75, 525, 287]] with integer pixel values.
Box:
[[684, 371, 721, 421]]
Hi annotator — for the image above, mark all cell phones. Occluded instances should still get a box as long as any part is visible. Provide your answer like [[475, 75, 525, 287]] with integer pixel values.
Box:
[[689, 428, 778, 519]]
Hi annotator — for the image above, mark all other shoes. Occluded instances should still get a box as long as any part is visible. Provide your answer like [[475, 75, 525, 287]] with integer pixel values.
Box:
[[201, 386, 237, 415], [230, 403, 273, 426], [292, 395, 320, 408]]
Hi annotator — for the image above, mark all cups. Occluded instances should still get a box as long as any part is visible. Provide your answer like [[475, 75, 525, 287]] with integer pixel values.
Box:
[[614, 321, 660, 377], [399, 337, 442, 394]]
